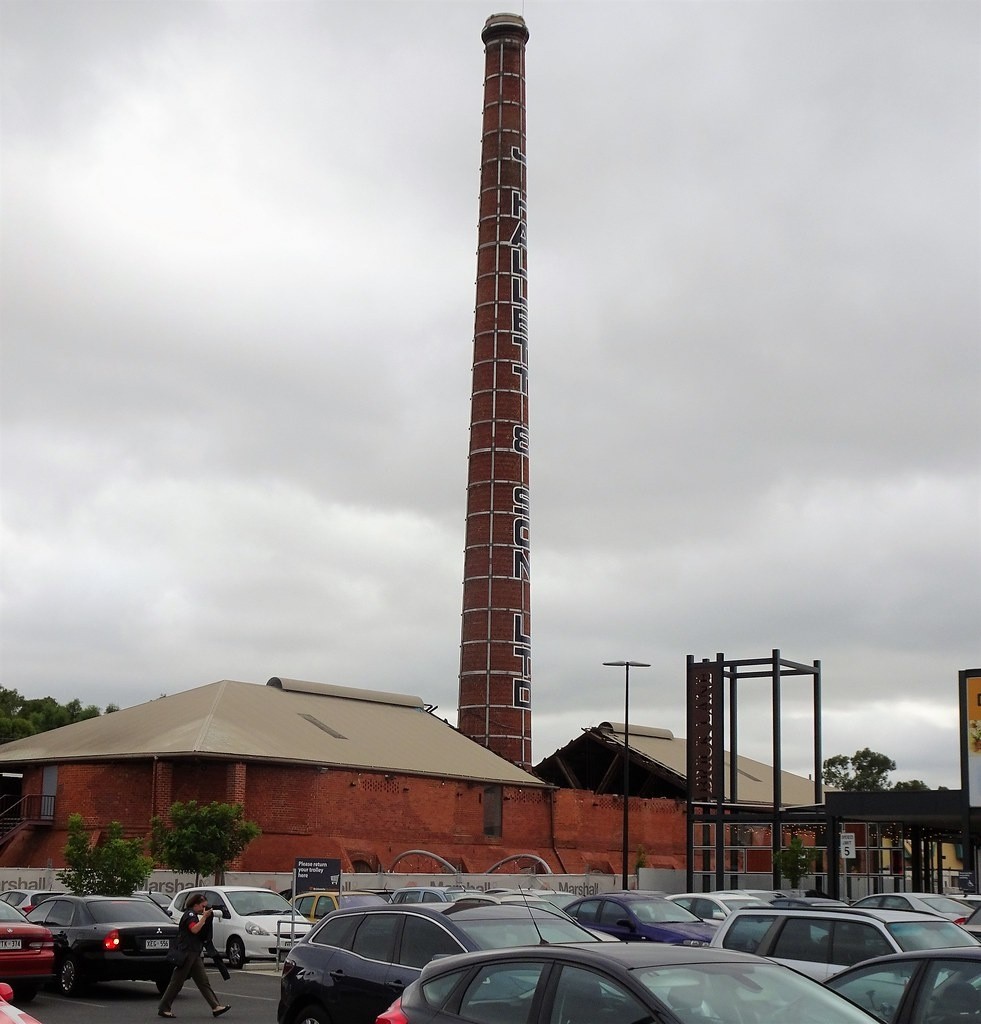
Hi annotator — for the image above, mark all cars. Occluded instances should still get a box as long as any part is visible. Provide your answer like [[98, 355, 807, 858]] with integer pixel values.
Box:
[[0, 886, 981, 1024]]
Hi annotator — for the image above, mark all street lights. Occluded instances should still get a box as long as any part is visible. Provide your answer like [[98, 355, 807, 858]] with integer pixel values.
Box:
[[600, 660, 653, 893]]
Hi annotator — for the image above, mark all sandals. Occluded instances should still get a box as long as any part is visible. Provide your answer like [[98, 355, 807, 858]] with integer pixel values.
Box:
[[158, 1010, 176, 1018], [213, 1005, 231, 1017]]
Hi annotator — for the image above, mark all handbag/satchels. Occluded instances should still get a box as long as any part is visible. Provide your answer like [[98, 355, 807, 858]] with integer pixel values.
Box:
[[165, 942, 190, 971]]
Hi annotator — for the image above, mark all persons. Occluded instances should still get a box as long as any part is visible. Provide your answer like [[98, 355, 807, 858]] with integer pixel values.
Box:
[[156, 894, 232, 1019]]
